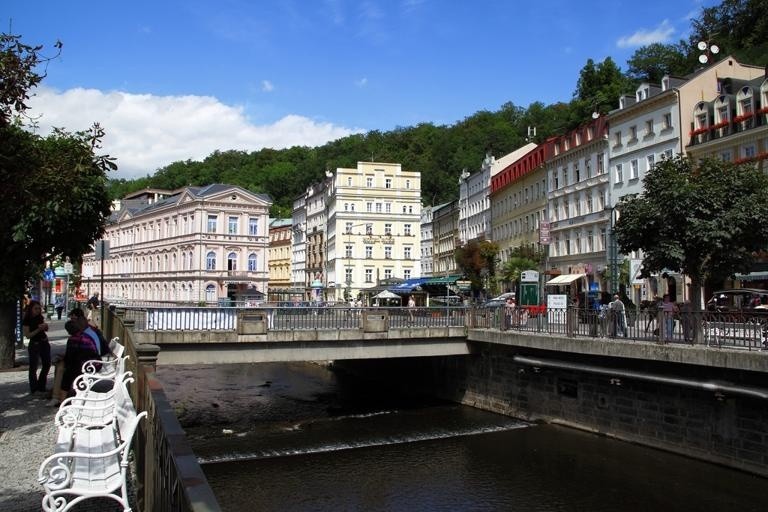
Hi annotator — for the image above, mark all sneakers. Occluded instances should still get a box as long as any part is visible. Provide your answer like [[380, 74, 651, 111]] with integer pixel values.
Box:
[[31, 389, 49, 399], [45, 399, 60, 407]]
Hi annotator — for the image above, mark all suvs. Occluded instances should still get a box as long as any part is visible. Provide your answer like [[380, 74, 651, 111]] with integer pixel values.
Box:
[[703, 288, 767, 338], [576, 291, 611, 324]]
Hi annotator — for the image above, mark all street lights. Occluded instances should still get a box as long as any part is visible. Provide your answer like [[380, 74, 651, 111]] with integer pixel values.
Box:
[[602, 201, 623, 297], [347, 221, 374, 305]]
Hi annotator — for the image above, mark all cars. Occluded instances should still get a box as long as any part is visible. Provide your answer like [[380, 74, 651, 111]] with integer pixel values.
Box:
[[276, 300, 339, 316], [476, 292, 515, 310]]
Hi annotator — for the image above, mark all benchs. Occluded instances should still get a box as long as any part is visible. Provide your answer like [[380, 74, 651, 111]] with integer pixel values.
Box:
[[37, 335, 149, 512]]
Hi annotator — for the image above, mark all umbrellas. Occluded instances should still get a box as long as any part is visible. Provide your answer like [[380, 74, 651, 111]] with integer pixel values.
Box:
[[371, 289, 402, 299]]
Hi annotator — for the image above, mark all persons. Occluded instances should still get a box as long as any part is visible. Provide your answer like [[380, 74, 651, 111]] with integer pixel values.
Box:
[[86, 291, 100, 322], [55, 295, 64, 320], [657, 293, 679, 341], [75, 316, 102, 356], [505, 298, 512, 330], [407, 295, 416, 323], [44, 320, 103, 409], [348, 299, 356, 318], [355, 298, 363, 317], [67, 306, 110, 357], [22, 300, 54, 396], [608, 294, 628, 338], [510, 298, 518, 328]]
